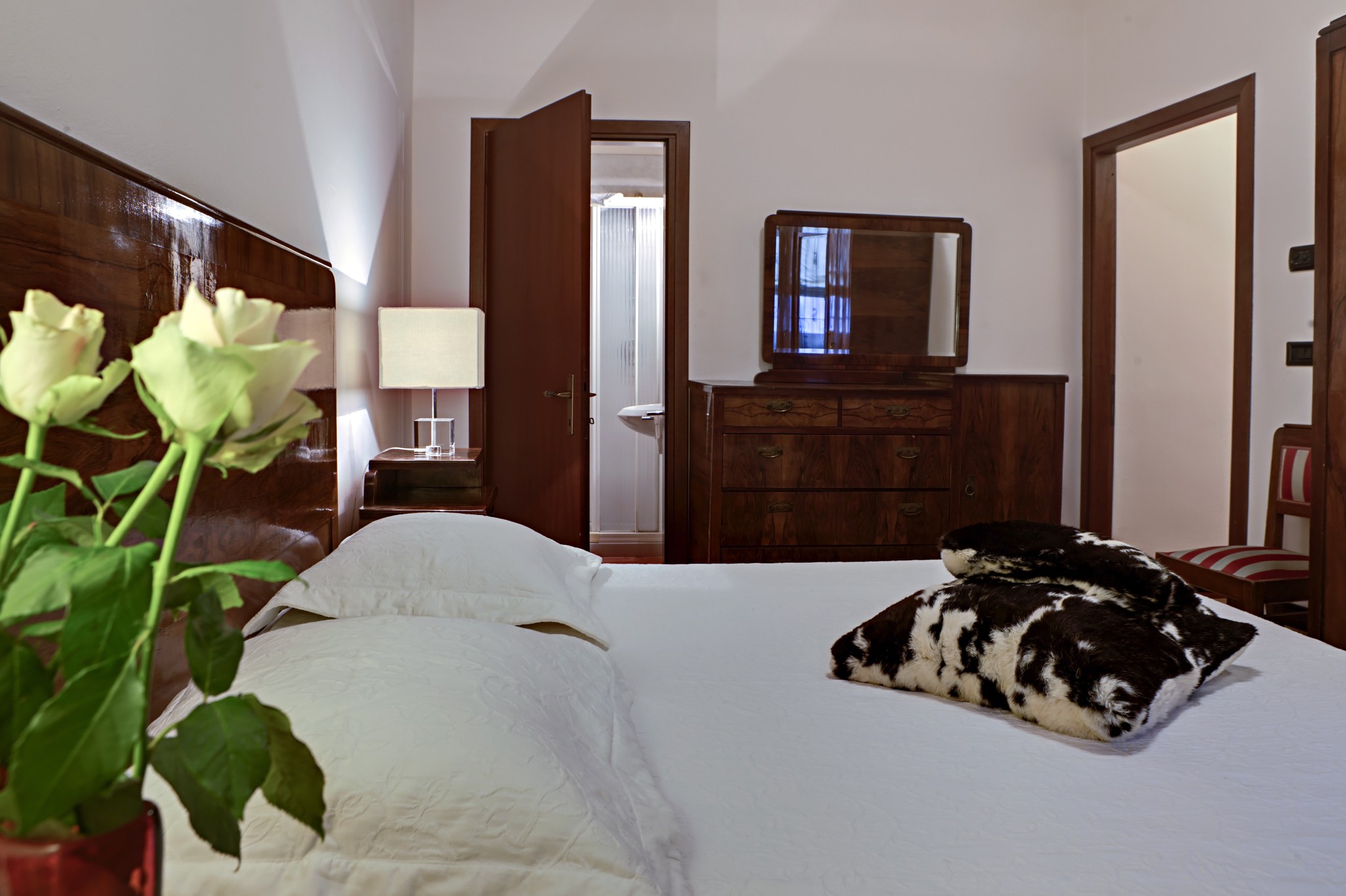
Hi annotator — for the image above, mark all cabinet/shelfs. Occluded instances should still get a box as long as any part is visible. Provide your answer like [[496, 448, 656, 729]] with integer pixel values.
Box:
[[682, 375, 1070, 551]]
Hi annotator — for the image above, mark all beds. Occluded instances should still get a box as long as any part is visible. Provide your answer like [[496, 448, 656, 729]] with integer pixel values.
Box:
[[0, 104, 1346, 896]]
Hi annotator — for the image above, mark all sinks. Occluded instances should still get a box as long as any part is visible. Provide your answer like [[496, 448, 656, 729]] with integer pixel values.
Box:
[[617, 403, 662, 416]]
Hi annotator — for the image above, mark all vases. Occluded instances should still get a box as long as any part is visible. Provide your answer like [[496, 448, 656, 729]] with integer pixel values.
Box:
[[0, 773, 165, 896]]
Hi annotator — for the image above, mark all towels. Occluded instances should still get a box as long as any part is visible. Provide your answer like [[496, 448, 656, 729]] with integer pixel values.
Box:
[[654, 415, 659, 454]]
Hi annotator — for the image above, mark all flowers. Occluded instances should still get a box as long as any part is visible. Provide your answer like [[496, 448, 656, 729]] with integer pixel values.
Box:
[[0, 282, 326, 874]]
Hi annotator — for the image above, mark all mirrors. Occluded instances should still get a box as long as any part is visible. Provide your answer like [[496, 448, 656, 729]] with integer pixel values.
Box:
[[762, 210, 973, 381]]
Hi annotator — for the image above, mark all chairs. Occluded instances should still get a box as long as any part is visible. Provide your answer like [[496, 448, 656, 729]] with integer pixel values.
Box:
[[1155, 424, 1318, 639]]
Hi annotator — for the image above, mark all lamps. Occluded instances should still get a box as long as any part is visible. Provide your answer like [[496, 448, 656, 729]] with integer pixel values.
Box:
[[378, 306, 487, 458]]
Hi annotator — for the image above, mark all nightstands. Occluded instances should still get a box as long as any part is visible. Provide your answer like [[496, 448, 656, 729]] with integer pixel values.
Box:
[[362, 443, 497, 518]]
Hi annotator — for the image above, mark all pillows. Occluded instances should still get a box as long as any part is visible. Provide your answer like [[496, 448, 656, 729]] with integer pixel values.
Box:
[[825, 515, 1258, 735], [114, 613, 696, 896], [240, 510, 612, 649]]
